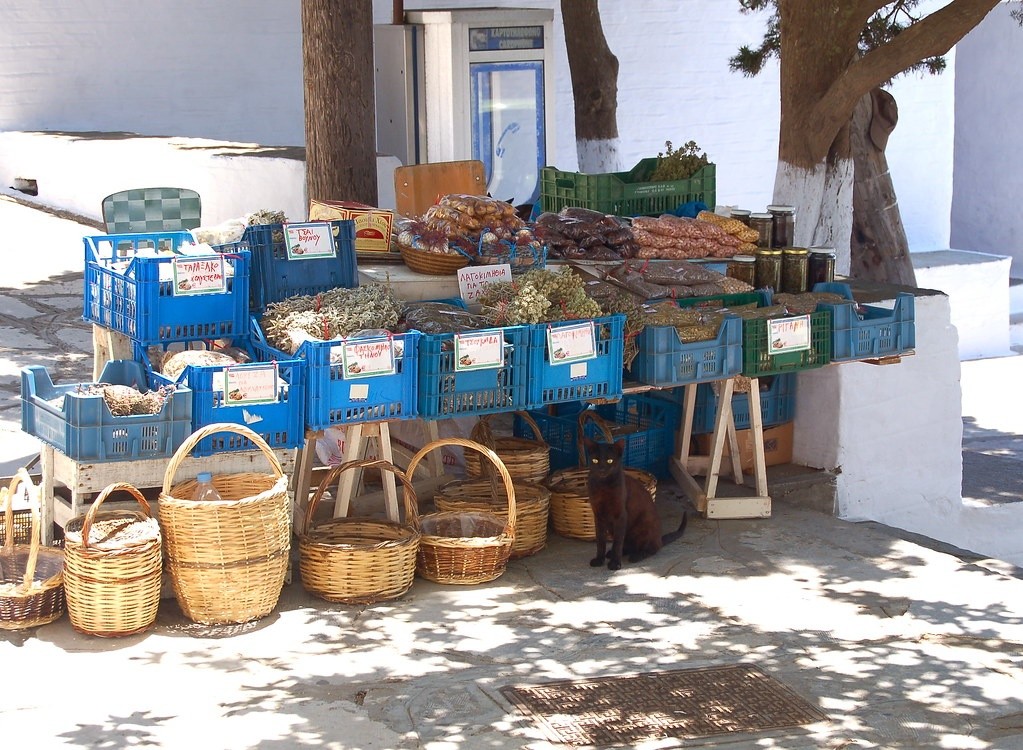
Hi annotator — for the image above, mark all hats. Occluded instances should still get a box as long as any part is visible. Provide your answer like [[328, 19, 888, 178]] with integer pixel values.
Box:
[[869, 88, 898, 152]]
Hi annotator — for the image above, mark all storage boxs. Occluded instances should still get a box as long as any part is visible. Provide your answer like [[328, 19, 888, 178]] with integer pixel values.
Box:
[[641, 373, 799, 435], [676, 424, 793, 482], [417, 298, 529, 422], [21, 359, 192, 464], [539, 157, 717, 218], [744, 311, 832, 379], [646, 291, 763, 308], [131, 340, 307, 458], [241, 218, 358, 316], [251, 311, 421, 430], [82, 231, 250, 346], [510, 395, 676, 481], [454, 283, 628, 411], [814, 282, 915, 362], [308, 200, 393, 252], [633, 315, 743, 386]]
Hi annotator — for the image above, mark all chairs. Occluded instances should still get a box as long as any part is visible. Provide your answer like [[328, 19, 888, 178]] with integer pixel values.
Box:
[[100, 187, 201, 256]]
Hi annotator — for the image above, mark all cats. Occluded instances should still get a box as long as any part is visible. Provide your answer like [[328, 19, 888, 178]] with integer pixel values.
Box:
[[581, 435, 688, 571]]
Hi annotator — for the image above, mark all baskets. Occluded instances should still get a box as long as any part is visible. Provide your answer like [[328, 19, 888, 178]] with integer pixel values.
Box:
[[158, 423, 292, 626], [474, 242, 553, 274], [433, 421, 550, 560], [399, 301, 486, 335], [403, 437, 517, 585], [0, 468, 65, 629], [394, 239, 475, 275], [463, 412, 550, 486], [547, 410, 658, 543], [64, 482, 162, 639], [298, 459, 421, 603]]
